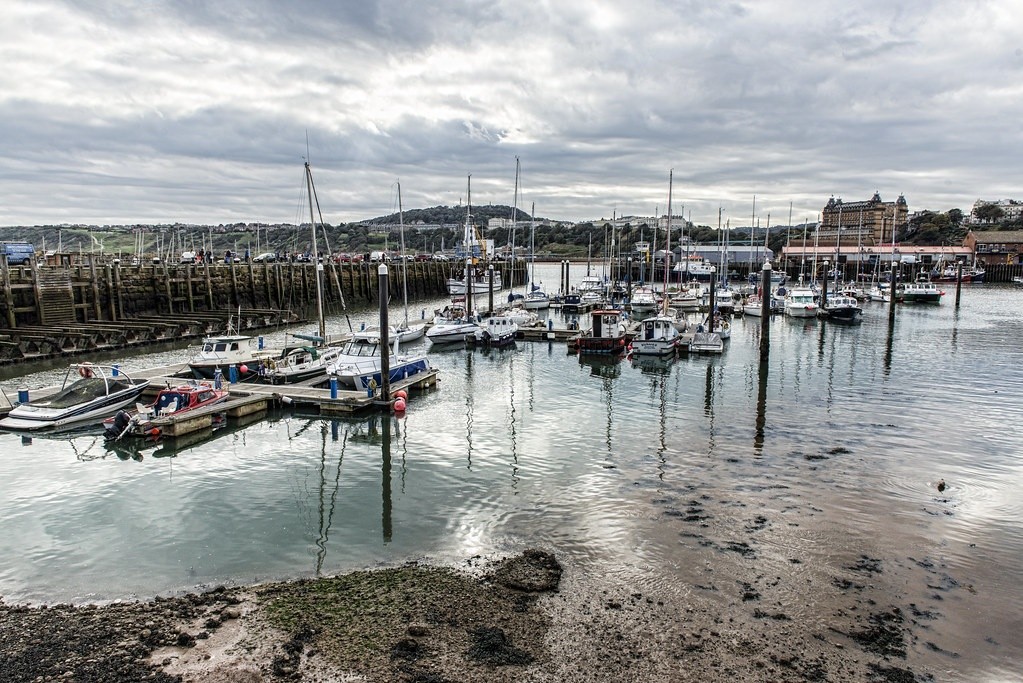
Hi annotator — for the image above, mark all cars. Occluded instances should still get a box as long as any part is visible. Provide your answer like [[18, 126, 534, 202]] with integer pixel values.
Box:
[[253, 253, 275, 263], [180, 251, 194, 264]]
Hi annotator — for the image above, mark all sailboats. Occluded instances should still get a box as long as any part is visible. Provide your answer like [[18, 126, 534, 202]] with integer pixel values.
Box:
[[345, 178, 428, 344], [256, 161, 355, 384], [428, 155, 990, 358]]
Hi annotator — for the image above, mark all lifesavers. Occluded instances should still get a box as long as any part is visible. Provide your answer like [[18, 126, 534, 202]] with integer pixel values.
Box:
[[177, 385, 193, 391], [81, 363, 93, 378]]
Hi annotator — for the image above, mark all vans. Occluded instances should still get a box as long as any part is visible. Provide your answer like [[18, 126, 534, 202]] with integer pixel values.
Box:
[[0, 241, 36, 267]]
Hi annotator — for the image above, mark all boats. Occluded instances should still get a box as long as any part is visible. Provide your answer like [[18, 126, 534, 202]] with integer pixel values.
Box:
[[0, 361, 152, 432], [101, 379, 231, 441], [325, 323, 430, 388], [188, 316, 284, 384]]
